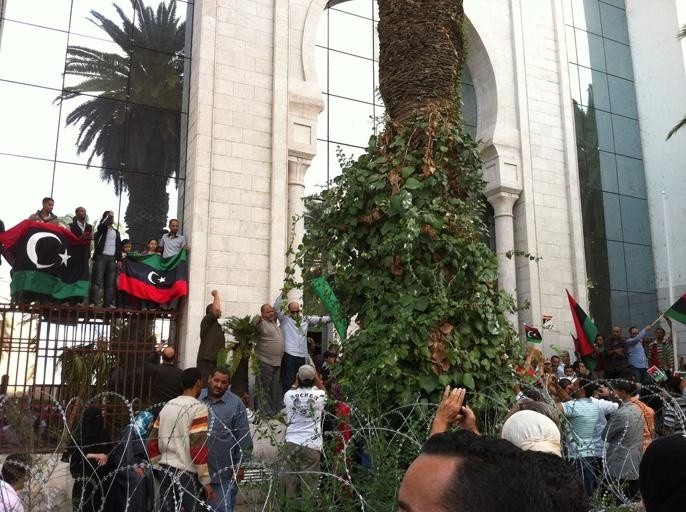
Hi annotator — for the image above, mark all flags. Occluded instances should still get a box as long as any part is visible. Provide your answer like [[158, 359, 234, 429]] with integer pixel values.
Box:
[[2, 217, 92, 303], [117, 248, 189, 303], [520, 280, 686, 405]]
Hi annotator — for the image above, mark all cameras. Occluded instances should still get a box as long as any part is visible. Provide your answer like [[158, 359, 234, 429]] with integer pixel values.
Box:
[[459, 402, 466, 416]]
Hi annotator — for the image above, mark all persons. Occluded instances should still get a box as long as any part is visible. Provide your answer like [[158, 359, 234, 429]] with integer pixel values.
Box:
[[0, 289, 356, 511], [32, 196, 187, 308], [395, 385, 686, 512]]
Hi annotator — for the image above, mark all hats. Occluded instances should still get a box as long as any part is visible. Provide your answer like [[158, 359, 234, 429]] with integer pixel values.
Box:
[[323, 351, 338, 359], [298, 364, 316, 382]]
